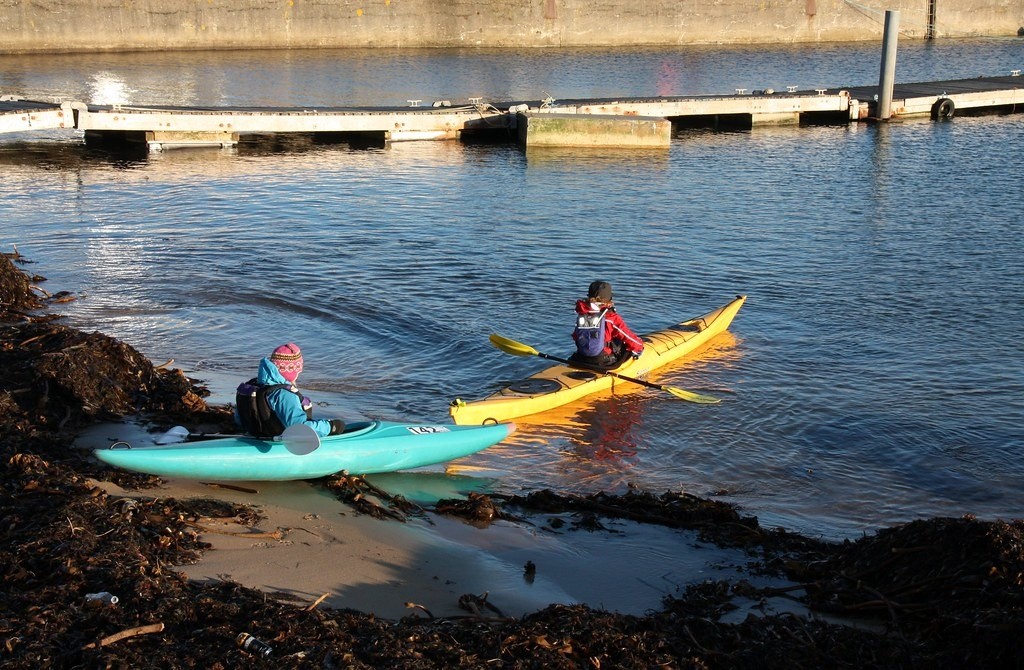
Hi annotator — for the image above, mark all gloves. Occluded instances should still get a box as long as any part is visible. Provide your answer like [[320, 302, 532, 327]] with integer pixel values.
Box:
[[329, 420, 345, 434]]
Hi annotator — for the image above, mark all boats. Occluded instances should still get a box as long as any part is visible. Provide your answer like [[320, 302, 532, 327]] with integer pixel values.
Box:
[[449, 294, 748, 426], [92, 421, 517, 482]]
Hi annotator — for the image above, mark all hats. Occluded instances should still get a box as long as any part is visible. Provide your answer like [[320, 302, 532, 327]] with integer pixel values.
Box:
[[270, 342, 303, 387], [587, 281, 612, 302]]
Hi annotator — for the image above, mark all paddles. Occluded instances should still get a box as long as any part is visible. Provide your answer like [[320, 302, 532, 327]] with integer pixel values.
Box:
[[488, 332, 723, 404], [156, 423, 322, 455]]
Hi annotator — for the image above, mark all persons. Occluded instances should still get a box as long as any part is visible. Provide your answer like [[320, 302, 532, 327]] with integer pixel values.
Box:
[[571, 279, 645, 368], [236, 343, 345, 436]]
